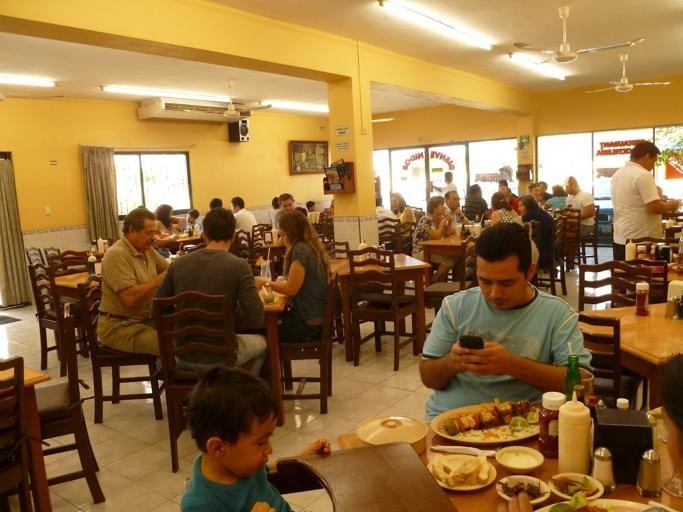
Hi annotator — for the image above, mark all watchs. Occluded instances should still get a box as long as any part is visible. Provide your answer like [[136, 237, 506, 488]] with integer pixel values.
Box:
[[265, 282, 270, 287]]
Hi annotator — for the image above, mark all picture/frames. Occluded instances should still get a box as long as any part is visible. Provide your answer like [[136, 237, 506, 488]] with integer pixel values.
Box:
[[288, 140, 328, 176]]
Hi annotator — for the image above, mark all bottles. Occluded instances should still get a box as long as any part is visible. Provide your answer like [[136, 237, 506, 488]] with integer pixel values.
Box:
[[637, 246, 646, 260], [558, 391, 590, 475], [636, 449, 661, 499], [617, 398, 628, 410], [625, 237, 637, 261], [635, 283, 650, 316], [566, 354, 581, 401], [104, 240, 108, 252], [537, 391, 566, 458], [359, 240, 367, 262], [650, 245, 656, 261], [188, 224, 192, 231], [665, 299, 678, 319], [195, 222, 200, 236], [98, 236, 104, 252], [597, 400, 606, 409], [574, 385, 585, 408], [585, 396, 596, 418], [366, 253, 370, 259], [91, 240, 97, 252], [647, 415, 657, 450], [88, 254, 97, 277], [376, 246, 385, 262], [675, 296, 681, 319], [592, 448, 615, 494]]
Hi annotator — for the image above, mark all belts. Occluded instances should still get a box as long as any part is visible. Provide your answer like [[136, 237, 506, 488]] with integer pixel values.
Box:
[[98, 310, 127, 320]]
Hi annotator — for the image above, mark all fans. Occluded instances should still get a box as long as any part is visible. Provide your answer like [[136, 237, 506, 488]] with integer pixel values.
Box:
[[513, 5, 630, 64], [192, 79, 272, 121], [584, 53, 671, 94]]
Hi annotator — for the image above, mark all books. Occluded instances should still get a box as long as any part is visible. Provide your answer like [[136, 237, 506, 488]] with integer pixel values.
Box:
[[324, 158, 351, 191]]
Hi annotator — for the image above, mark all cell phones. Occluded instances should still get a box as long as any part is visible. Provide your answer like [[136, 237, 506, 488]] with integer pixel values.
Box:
[[458, 333, 485, 350]]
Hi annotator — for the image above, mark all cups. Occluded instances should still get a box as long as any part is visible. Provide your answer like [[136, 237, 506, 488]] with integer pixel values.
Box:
[[474, 224, 482, 240], [454, 223, 462, 238], [261, 288, 277, 305], [260, 261, 272, 279]]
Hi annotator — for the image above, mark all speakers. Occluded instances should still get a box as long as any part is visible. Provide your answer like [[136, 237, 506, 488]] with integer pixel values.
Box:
[[228, 119, 239, 142], [239, 118, 250, 142]]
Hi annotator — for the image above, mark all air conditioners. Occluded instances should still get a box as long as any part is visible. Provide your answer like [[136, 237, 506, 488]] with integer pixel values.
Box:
[[138, 98, 252, 122]]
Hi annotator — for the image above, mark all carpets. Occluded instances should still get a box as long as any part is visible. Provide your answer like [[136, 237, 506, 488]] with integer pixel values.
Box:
[[0, 314, 22, 325]]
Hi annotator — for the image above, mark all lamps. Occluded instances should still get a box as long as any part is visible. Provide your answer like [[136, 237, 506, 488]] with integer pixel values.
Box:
[[378, 0, 497, 51], [258, 99, 329, 113], [0, 74, 60, 88], [508, 51, 572, 81], [100, 85, 236, 104]]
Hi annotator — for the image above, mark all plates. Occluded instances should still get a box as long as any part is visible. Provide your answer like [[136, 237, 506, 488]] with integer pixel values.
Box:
[[431, 402, 543, 447], [533, 499, 665, 512], [425, 455, 497, 493]]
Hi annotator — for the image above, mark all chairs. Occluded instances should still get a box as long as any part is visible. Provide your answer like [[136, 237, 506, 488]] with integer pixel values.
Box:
[[44, 246, 61, 267], [554, 208, 581, 272], [28, 262, 91, 377], [346, 247, 419, 371], [315, 212, 328, 241], [252, 223, 272, 249], [35, 303, 106, 504], [531, 215, 567, 296], [232, 229, 255, 277], [46, 260, 88, 358], [378, 218, 401, 250], [578, 314, 647, 411], [264, 270, 339, 414], [0, 355, 33, 512], [47, 249, 91, 277], [578, 260, 653, 312], [611, 258, 668, 308], [396, 221, 418, 257], [424, 235, 478, 334], [26, 246, 45, 265], [154, 290, 236, 473], [167, 236, 201, 249], [326, 241, 351, 261], [626, 237, 670, 259], [665, 227, 683, 245], [77, 274, 165, 424]]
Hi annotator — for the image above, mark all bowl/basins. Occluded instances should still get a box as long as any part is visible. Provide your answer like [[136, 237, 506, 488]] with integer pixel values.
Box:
[[496, 475, 550, 505], [548, 472, 604, 500], [356, 414, 427, 457], [495, 446, 544, 473]]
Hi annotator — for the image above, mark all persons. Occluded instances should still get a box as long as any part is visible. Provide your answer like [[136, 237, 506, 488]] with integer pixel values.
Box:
[[517, 194, 555, 268], [153, 204, 186, 237], [230, 196, 264, 246], [256, 209, 331, 343], [565, 175, 595, 241], [498, 179, 520, 198], [528, 182, 548, 209], [412, 196, 457, 283], [199, 198, 223, 235], [269, 197, 280, 230], [496, 353, 683, 512], [490, 191, 524, 226], [539, 181, 553, 201], [433, 172, 458, 196], [444, 191, 472, 231], [419, 222, 595, 423], [95, 208, 171, 371], [149, 207, 268, 378], [544, 185, 567, 209], [464, 184, 490, 220], [181, 209, 203, 234], [306, 200, 316, 212], [390, 192, 417, 247], [180, 366, 331, 512], [487, 189, 521, 218], [376, 197, 397, 249], [273, 193, 300, 229], [610, 140, 680, 260]]
